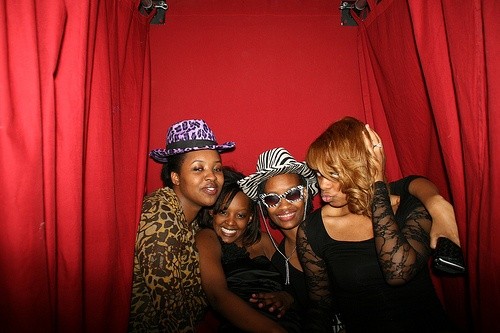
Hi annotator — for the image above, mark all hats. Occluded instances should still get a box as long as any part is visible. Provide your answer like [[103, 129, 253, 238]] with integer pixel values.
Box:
[[149, 118, 235, 164], [236, 147, 319, 285]]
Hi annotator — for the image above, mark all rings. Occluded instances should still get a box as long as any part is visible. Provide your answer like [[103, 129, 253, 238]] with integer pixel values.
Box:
[[373, 142, 381, 149]]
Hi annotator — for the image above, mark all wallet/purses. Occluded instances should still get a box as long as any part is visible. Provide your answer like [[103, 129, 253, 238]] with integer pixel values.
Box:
[[429, 236, 466, 276]]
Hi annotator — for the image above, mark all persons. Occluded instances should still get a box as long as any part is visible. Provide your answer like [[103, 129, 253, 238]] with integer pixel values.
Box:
[[295, 117, 449, 333], [238, 148, 460, 333], [129, 119, 236, 333], [191, 166, 294, 333]]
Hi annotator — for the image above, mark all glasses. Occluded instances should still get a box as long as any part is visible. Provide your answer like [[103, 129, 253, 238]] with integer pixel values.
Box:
[[258, 184, 307, 208]]
